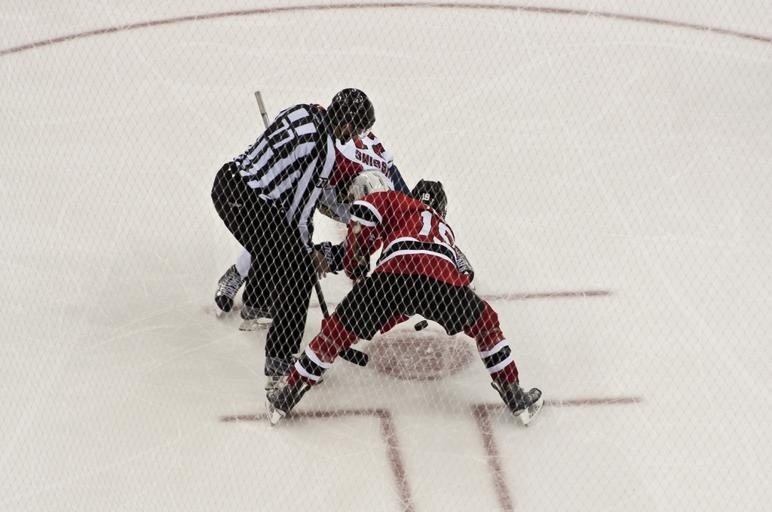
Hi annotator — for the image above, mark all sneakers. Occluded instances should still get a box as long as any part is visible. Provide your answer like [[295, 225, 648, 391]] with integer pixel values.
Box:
[[265, 354, 310, 414], [215, 264, 274, 319], [506, 383, 541, 415]]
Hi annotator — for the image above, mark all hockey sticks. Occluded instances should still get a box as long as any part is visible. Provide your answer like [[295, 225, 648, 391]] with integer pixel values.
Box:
[[255, 91, 367, 366]]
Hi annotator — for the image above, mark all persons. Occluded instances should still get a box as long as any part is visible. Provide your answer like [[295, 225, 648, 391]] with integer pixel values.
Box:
[[267, 178, 542, 420], [214, 128, 411, 317], [213, 86, 376, 398]]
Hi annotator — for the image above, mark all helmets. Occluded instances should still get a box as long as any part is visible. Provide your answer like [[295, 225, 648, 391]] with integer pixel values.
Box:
[[327, 88, 375, 144], [347, 170, 395, 201], [412, 178, 447, 220]]
